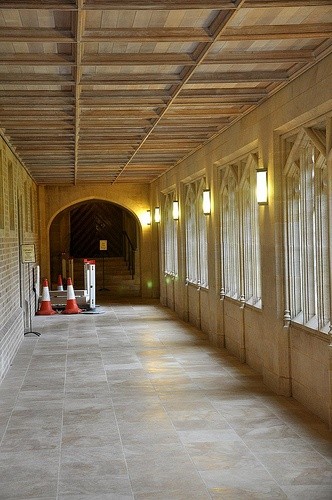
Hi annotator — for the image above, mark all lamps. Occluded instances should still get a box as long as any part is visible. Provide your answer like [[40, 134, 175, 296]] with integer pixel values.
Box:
[[255, 167, 268, 204], [146, 210, 151, 225], [155, 207, 160, 222], [202, 189, 210, 215], [172, 201, 179, 220]]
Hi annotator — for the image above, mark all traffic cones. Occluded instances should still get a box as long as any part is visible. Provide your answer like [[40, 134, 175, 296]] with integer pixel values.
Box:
[[61, 277, 85, 313], [56, 274, 63, 291], [35, 279, 57, 315]]
